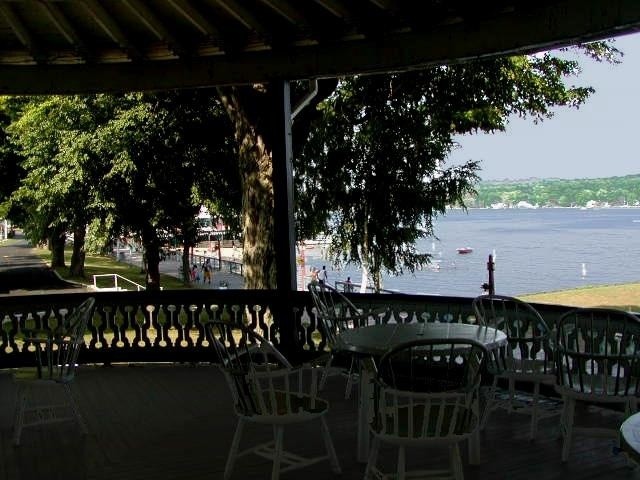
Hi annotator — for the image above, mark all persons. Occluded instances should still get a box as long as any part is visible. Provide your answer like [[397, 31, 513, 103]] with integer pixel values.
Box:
[[190, 257, 213, 285], [316, 265, 328, 291], [343, 277, 353, 291]]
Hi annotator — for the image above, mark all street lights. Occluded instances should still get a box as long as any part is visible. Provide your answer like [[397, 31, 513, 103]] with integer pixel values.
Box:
[[215, 233, 222, 271]]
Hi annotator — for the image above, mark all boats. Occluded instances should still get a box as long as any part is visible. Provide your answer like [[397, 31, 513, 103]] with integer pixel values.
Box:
[[455, 246, 475, 254]]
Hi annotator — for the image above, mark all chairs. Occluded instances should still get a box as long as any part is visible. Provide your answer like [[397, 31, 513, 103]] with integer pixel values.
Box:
[[12, 296, 96, 446], [206, 282, 640, 478]]
